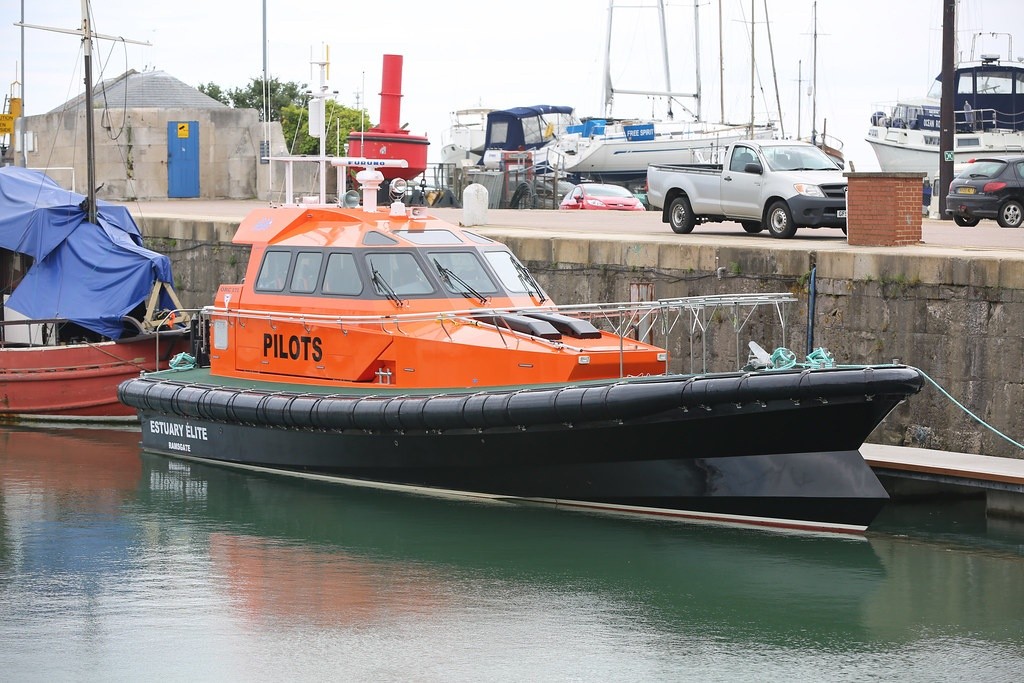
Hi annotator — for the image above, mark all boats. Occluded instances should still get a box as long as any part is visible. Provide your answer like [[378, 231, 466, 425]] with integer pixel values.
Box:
[[865, 32, 1024, 215], [441, 0, 845, 205], [0, 0, 199, 434], [115, 203, 926, 543]]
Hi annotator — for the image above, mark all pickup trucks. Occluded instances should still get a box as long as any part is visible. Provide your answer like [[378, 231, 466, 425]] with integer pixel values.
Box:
[[644, 139, 849, 239]]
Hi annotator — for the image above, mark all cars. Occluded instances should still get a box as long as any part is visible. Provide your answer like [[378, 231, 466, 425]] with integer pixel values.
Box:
[[944, 156, 1024, 228], [559, 182, 646, 211]]
